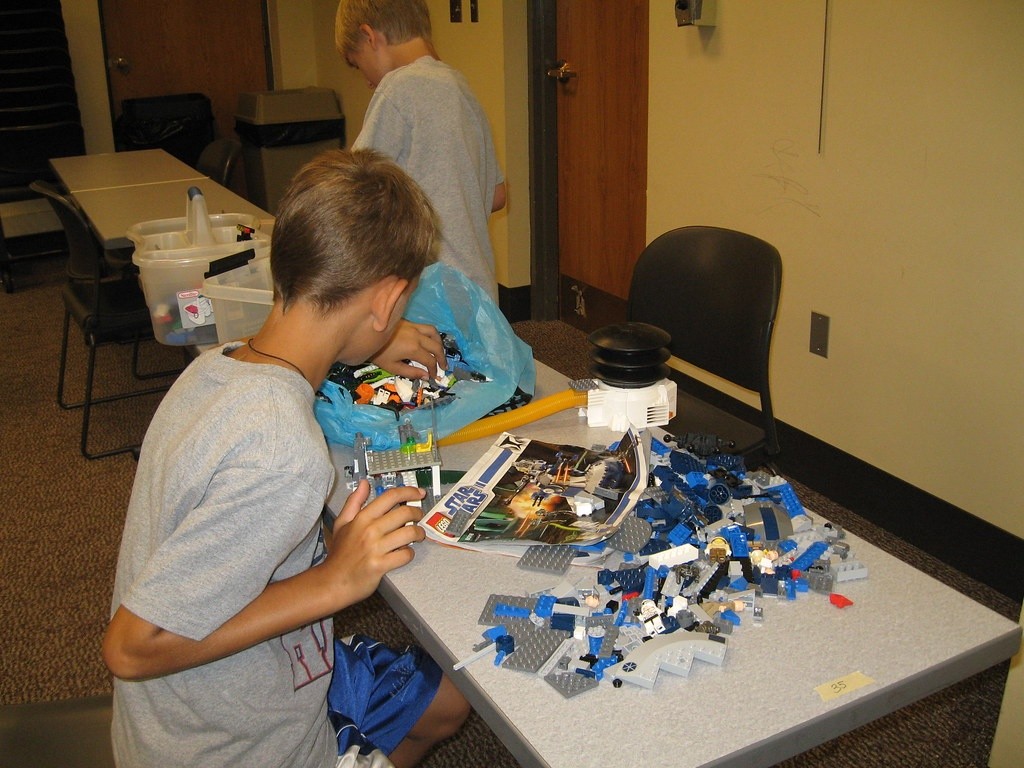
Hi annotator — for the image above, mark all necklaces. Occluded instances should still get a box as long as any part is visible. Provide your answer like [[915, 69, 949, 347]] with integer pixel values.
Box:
[[248, 338, 306, 379]]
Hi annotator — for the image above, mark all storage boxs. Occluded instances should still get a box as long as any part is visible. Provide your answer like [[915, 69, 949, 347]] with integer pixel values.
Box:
[[199, 255, 276, 344], [128, 213, 272, 347]]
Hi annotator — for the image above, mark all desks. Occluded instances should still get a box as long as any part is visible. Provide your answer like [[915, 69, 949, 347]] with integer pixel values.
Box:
[[49, 150, 1024, 768]]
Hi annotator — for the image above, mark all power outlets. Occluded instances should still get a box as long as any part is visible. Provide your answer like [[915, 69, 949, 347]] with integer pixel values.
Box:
[[809, 311, 830, 359]]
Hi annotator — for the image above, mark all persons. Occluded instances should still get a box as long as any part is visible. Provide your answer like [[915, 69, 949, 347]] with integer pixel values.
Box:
[[335, 0, 506, 308], [100, 149, 471, 768]]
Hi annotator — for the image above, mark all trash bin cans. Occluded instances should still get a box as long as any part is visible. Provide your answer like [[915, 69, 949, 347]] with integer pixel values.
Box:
[[234, 86, 345, 216], [121, 92, 213, 165]]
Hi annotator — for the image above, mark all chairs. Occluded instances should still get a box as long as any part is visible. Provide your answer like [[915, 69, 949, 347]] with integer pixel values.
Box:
[[626, 225, 786, 478], [196, 136, 242, 189], [31, 181, 187, 459]]
